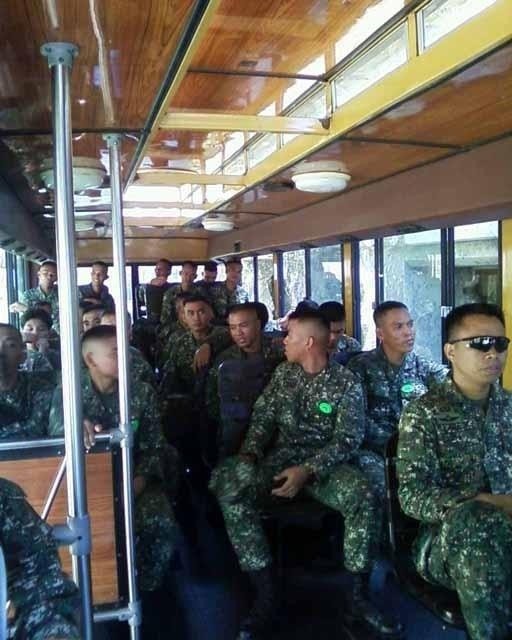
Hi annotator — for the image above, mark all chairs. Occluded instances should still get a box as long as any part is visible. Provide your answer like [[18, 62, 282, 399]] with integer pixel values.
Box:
[[373, 431, 463, 630], [218, 356, 340, 564]]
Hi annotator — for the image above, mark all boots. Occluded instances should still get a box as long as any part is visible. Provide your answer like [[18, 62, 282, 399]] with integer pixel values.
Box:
[[236, 560, 281, 640], [342, 569, 405, 635]]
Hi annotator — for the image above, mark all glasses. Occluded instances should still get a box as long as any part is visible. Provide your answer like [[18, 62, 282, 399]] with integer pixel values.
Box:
[[451, 335, 508, 354]]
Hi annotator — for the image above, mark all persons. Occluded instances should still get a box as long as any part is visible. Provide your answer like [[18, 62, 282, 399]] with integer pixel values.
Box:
[[166, 261, 204, 294], [220, 261, 250, 304], [83, 304, 105, 331], [195, 261, 222, 298], [31, 300, 54, 317], [0, 322, 57, 461], [209, 310, 406, 640], [156, 298, 237, 475], [150, 293, 200, 372], [78, 262, 115, 308], [397, 303, 511, 640], [50, 326, 185, 615], [149, 258, 172, 289], [210, 306, 288, 469], [347, 301, 454, 550], [319, 299, 362, 366], [0, 476, 84, 640], [7, 262, 59, 313], [19, 308, 60, 370], [100, 308, 132, 339]]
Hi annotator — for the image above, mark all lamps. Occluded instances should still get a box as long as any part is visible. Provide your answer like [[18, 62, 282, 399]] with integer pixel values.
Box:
[[202, 213, 238, 233], [75, 218, 98, 234], [35, 155, 104, 189], [290, 163, 351, 196]]
